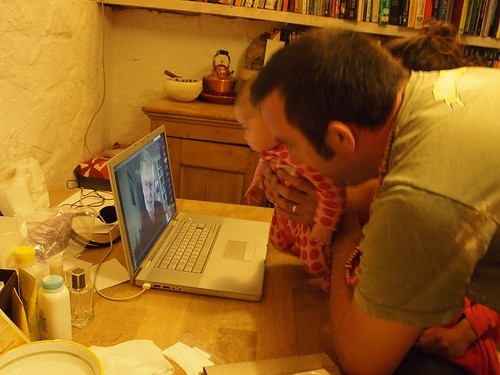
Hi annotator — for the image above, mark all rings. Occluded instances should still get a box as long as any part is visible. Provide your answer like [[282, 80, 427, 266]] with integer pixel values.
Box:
[[293, 204, 296, 213]]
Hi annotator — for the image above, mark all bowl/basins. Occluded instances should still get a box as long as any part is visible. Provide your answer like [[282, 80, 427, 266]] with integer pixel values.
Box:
[[163, 77, 202, 103]]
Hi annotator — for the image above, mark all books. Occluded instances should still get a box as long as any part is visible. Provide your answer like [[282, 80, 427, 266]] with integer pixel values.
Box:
[[200, 0, 500, 39], [281, 24, 382, 46], [464, 47, 500, 67]]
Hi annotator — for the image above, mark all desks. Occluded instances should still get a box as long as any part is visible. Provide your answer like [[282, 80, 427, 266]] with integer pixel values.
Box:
[[43, 190, 337, 374]]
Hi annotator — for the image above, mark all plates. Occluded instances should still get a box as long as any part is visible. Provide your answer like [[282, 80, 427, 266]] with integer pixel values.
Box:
[[0, 341, 103, 375], [202, 94, 236, 105]]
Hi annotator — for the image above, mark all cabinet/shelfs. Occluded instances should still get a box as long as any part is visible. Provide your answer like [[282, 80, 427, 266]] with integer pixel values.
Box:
[[141, 94, 271, 206]]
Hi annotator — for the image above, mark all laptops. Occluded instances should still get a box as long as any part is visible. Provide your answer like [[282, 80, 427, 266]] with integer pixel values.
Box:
[[108, 126, 275, 301]]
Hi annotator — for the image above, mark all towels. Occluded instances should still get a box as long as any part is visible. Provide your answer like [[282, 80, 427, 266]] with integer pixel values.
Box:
[[89, 337, 175, 375]]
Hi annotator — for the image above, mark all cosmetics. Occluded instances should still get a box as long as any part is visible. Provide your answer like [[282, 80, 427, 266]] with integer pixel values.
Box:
[[11, 245, 95, 342]]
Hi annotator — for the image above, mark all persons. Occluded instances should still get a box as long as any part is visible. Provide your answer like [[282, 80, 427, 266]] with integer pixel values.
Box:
[[250, 27, 500, 375], [234, 74, 348, 293], [134, 149, 167, 260], [387, 25, 500, 375]]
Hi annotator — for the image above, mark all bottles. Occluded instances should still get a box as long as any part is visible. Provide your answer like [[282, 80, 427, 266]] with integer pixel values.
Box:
[[14, 244, 94, 341]]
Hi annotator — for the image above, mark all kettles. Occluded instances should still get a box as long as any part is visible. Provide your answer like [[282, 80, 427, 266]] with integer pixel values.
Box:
[[202, 49, 238, 94]]
